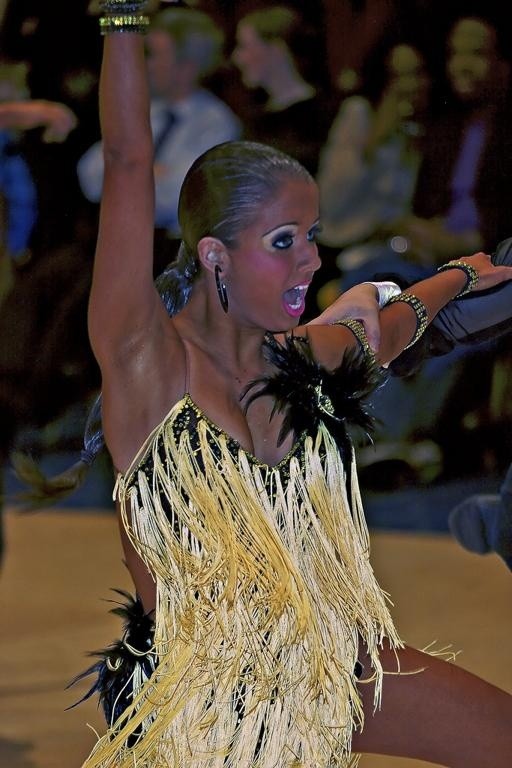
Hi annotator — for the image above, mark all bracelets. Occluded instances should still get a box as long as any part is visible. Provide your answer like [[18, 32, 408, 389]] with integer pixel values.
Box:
[[435, 258, 480, 301], [97, 0, 154, 37]]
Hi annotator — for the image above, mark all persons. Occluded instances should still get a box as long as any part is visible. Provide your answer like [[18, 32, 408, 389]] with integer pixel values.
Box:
[[83, 1, 512, 768]]
